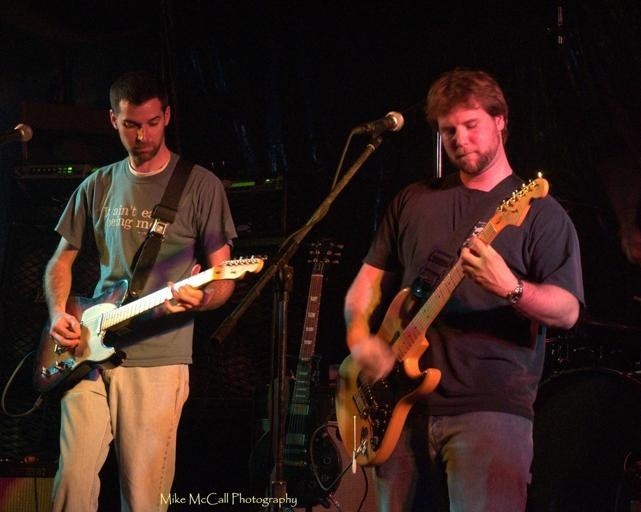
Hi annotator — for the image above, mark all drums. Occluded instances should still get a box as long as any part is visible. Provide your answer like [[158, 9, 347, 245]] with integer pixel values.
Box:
[[526, 366, 640, 512], [551, 314, 640, 382]]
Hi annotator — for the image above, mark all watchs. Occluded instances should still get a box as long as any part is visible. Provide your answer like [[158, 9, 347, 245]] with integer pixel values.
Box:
[[505, 276, 524, 305]]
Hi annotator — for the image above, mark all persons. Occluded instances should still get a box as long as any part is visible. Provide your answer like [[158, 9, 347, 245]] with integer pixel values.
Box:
[[344, 69, 586, 511], [43, 69, 239, 512]]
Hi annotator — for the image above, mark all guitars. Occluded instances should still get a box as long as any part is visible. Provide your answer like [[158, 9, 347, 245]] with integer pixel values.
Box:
[[33, 255, 268, 397], [335, 170, 551, 468], [248, 237, 343, 512]]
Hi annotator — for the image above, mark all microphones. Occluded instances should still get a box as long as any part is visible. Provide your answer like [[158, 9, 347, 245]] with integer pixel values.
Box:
[[363, 113, 407, 136]]
